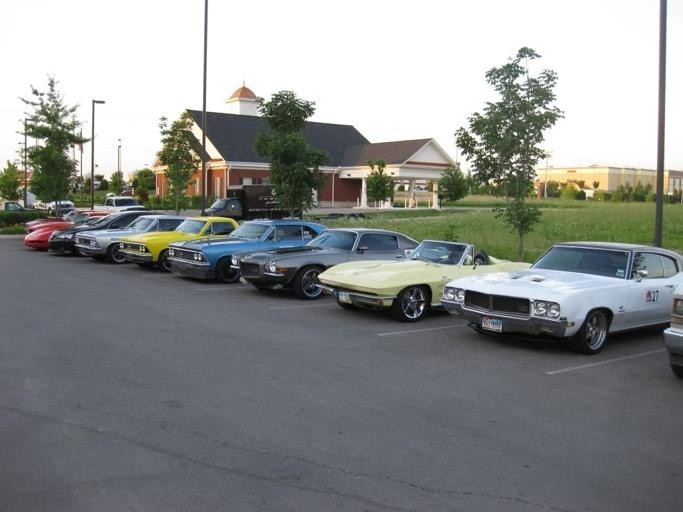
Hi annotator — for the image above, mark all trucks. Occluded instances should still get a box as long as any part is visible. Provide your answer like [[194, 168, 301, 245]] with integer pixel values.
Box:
[[198, 180, 299, 223]]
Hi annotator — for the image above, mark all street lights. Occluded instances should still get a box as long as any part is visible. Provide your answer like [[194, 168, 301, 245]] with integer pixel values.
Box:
[[90, 97, 107, 210], [543, 151, 552, 199], [117, 145, 122, 191], [23, 117, 35, 207]]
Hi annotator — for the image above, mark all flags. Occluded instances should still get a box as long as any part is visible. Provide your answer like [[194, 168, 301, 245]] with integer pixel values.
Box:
[[77, 133, 83, 153]]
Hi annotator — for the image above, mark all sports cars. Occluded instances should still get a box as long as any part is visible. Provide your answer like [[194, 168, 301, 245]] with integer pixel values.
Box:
[[313, 237, 534, 326]]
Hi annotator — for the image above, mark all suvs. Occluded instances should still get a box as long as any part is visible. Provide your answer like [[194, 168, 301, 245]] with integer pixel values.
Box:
[[71, 214, 190, 266]]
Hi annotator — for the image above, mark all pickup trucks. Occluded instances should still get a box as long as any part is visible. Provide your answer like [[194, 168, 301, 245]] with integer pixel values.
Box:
[[94, 195, 146, 212]]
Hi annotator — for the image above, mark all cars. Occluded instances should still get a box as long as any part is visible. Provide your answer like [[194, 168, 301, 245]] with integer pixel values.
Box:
[[30, 196, 75, 215], [1, 200, 30, 211], [661, 276, 681, 382], [232, 225, 422, 304], [166, 220, 327, 285], [435, 239, 682, 359], [20, 210, 114, 254], [118, 216, 239, 273]]
